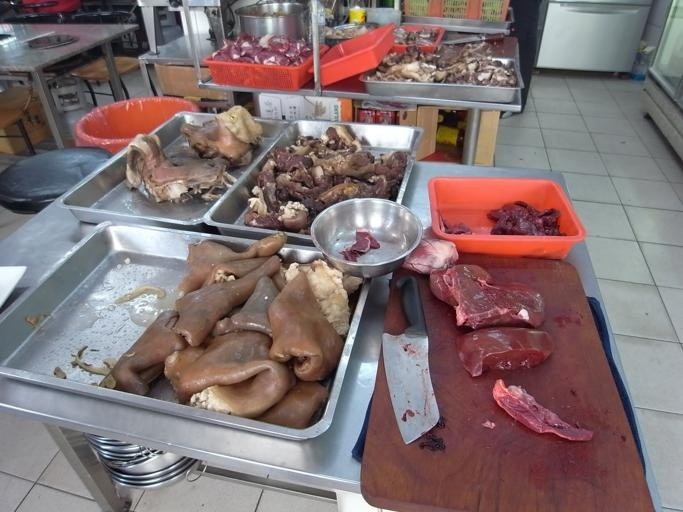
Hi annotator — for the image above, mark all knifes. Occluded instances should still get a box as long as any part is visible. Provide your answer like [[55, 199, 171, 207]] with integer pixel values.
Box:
[[379, 275, 441, 445], [441, 31, 506, 46]]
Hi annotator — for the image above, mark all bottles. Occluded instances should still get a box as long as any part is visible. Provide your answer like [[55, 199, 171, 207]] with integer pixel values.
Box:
[[307, 0, 325, 42], [631, 51, 649, 81]]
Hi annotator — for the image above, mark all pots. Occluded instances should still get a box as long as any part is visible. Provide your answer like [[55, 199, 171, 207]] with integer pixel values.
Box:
[[234, 0, 311, 49], [81, 430, 208, 493]]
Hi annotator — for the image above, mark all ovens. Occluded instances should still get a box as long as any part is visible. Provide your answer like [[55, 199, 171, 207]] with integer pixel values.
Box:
[[536, 1, 653, 80]]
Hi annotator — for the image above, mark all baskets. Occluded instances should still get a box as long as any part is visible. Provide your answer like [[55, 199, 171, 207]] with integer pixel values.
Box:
[[202, 42, 329, 92], [404, 0, 513, 22]]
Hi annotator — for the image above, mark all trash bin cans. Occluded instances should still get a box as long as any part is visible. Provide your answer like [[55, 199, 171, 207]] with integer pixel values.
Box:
[[74, 96, 201, 156]]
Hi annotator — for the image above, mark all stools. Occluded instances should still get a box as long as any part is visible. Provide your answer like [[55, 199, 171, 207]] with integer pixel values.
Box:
[[0, 84, 40, 157], [69, 53, 157, 109]]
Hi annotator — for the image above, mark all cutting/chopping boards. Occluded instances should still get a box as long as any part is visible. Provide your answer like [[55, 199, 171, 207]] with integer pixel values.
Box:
[[358, 253, 658, 511]]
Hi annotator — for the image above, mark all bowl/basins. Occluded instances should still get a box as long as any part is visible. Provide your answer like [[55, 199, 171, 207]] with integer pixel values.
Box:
[[309, 197, 425, 281], [366, 6, 402, 28], [325, 21, 380, 46]]
